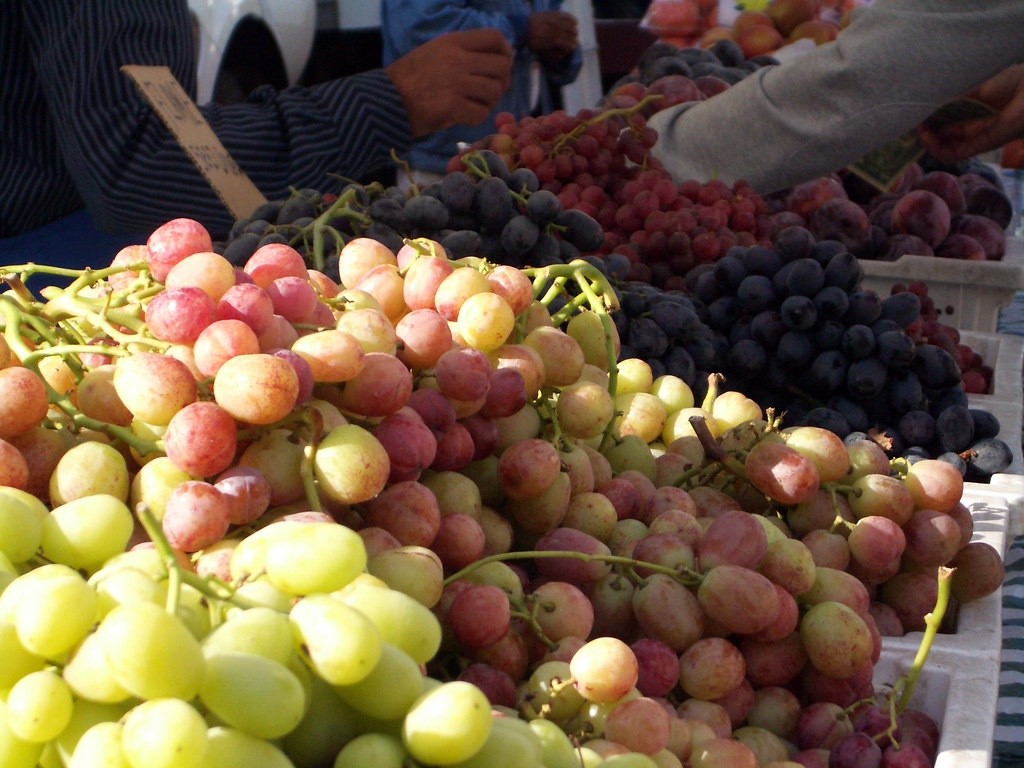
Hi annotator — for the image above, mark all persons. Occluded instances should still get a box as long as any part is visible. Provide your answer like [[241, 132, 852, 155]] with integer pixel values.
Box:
[[620, 0, 1024, 201], [369, 0, 584, 185], [0, 0, 513, 303]]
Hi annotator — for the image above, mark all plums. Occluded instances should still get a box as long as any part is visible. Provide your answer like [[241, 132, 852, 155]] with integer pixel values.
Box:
[[601, 37, 1013, 263]]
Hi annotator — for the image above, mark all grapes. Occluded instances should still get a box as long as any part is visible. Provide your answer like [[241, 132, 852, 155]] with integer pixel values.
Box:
[[0, 218, 1006, 768], [447, 92, 776, 292], [224, 149, 1015, 479]]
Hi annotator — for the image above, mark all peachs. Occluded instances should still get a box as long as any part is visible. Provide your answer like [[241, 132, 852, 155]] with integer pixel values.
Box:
[[644, 0, 860, 55]]
